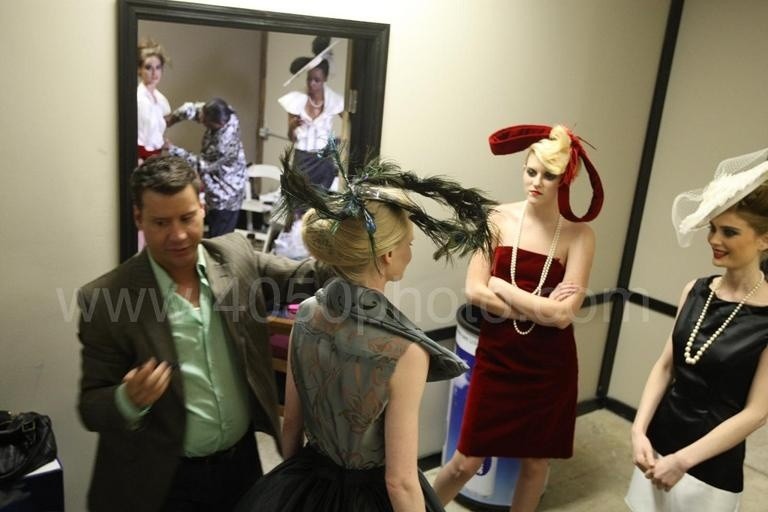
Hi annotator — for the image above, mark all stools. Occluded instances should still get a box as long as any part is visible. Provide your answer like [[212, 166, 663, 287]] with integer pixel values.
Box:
[[261, 304, 299, 416]]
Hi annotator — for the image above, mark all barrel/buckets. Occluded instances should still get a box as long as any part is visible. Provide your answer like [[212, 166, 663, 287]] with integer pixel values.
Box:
[[442, 307, 550, 507]]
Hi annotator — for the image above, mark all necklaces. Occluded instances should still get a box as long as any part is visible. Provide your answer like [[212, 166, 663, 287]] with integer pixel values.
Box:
[[309, 95, 323, 116], [511, 199, 565, 336], [684, 270, 765, 365]]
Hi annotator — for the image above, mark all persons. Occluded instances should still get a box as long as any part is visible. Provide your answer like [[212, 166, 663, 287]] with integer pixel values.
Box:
[[278, 59, 344, 219], [137, 41, 248, 238], [623, 148, 768, 512], [432, 126, 595, 512]]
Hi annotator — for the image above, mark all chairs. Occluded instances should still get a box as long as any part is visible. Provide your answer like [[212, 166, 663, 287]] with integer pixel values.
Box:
[[232, 164, 281, 254]]
